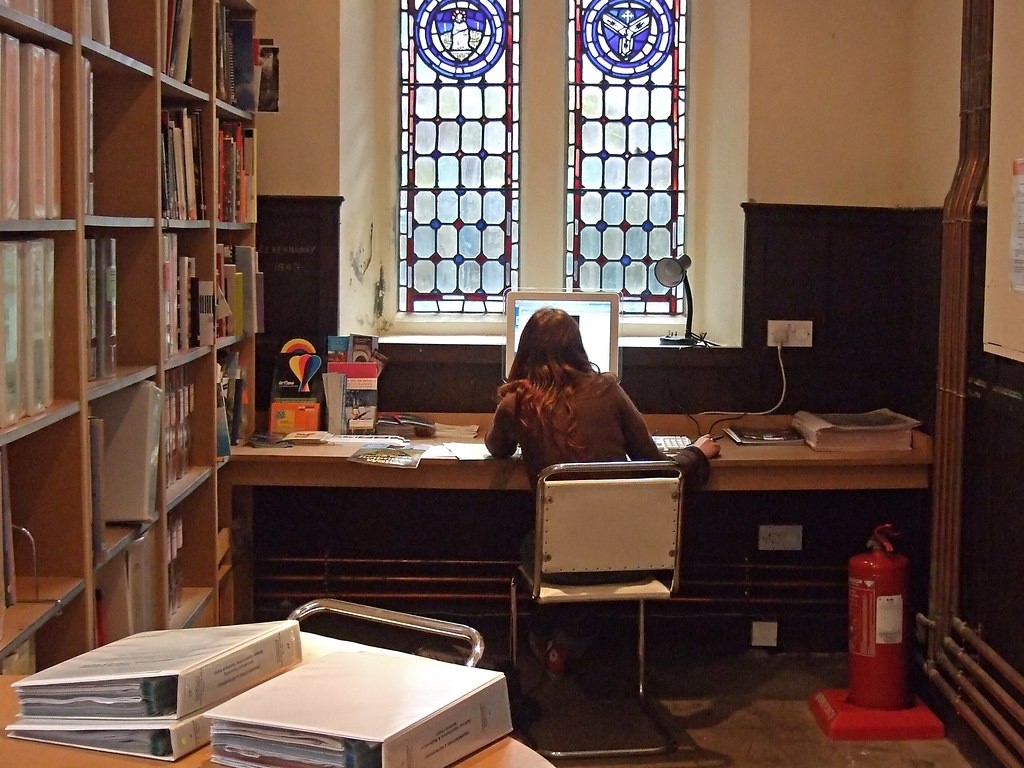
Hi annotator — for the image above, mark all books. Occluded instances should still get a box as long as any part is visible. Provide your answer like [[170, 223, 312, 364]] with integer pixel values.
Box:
[[724, 426, 805, 445], [0, 0, 514, 768]]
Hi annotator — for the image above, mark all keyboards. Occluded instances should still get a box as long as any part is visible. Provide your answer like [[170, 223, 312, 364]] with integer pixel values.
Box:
[[651, 436, 691, 454]]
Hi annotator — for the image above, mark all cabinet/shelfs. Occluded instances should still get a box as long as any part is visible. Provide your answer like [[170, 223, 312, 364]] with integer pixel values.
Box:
[[0, 0, 259, 675]]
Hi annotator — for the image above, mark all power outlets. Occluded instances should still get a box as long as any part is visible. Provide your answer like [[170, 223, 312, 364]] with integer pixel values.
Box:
[[767, 320, 813, 348], [758, 524, 803, 551]]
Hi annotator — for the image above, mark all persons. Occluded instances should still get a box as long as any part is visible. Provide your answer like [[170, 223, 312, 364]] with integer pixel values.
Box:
[[486, 307, 721, 682]]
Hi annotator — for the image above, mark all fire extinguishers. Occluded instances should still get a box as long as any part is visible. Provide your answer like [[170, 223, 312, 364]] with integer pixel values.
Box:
[[846, 523, 915, 711]]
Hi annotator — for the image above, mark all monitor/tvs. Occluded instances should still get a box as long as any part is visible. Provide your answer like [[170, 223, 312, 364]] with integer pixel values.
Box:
[[501, 287, 622, 384]]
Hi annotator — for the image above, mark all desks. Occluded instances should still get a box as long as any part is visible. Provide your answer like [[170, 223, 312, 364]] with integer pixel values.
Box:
[[230, 412, 934, 490], [0, 675, 556, 768]]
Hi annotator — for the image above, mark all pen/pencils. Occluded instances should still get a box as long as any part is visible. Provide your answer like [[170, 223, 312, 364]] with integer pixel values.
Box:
[[691, 435, 724, 444]]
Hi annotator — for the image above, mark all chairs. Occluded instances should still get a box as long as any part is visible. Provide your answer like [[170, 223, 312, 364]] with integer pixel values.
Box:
[[510, 460, 684, 760]]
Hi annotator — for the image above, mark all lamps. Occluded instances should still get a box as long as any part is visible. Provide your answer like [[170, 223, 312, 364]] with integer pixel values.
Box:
[[654, 255, 698, 346]]
